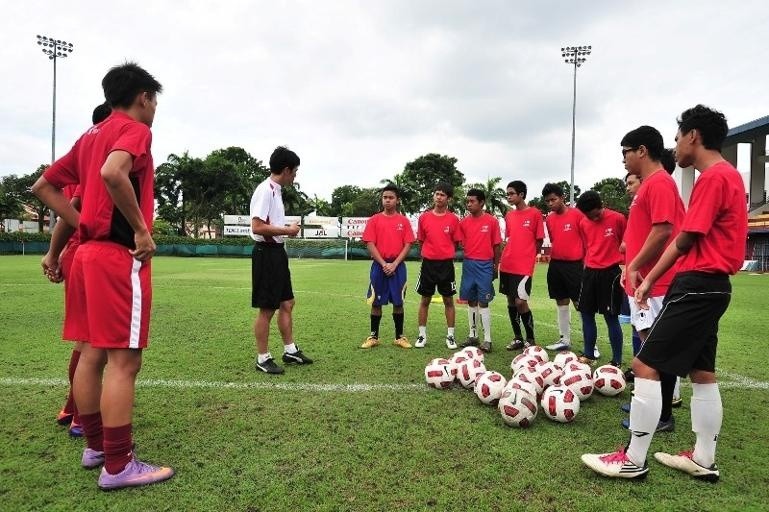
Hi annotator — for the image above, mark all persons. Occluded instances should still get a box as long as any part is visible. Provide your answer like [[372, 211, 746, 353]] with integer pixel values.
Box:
[[359, 183, 413, 349], [576, 190, 628, 369], [249, 146, 313, 373], [581, 104, 748, 485], [499, 180, 545, 352], [414, 184, 465, 350], [459, 188, 504, 354], [32, 62, 174, 492], [617, 148, 686, 409], [40, 101, 114, 435], [542, 183, 600, 359], [619, 125, 678, 435]]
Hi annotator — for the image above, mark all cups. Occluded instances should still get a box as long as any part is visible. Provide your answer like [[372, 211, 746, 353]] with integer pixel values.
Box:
[[622, 148, 639, 158]]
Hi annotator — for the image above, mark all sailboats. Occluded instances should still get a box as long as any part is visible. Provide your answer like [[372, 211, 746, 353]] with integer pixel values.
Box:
[[424, 346, 627, 429]]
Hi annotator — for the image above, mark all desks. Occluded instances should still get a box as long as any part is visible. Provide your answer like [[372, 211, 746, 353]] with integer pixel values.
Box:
[[36, 33, 74, 237], [561, 44, 593, 209]]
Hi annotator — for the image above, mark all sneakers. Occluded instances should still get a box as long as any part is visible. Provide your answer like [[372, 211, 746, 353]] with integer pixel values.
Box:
[[393, 337, 412, 349], [282, 344, 313, 365], [256, 358, 285, 374], [506, 339, 524, 351], [672, 394, 682, 407], [581, 448, 649, 480], [445, 336, 458, 350], [56, 405, 174, 492], [546, 339, 570, 351], [460, 337, 481, 348], [578, 354, 595, 364], [624, 368, 635, 383], [655, 452, 718, 480], [414, 336, 427, 347], [622, 402, 675, 433], [363, 336, 380, 349], [522, 340, 536, 351], [480, 341, 492, 353], [609, 361, 622, 369]]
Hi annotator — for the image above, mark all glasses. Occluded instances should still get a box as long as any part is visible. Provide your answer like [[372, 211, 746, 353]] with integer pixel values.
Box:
[[622, 148, 639, 158]]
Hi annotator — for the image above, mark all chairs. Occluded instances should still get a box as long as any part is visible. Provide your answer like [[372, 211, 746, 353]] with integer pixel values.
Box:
[[56, 405, 174, 492], [256, 358, 285, 374], [282, 344, 313, 365]]
